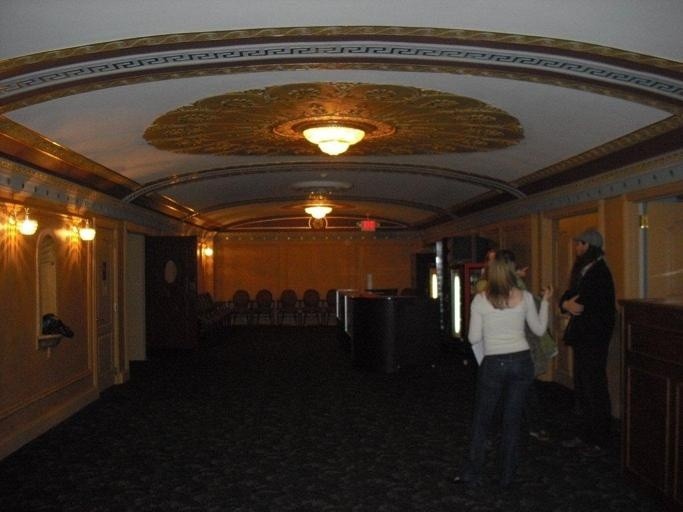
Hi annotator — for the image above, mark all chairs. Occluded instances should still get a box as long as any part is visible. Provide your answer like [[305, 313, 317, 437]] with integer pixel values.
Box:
[[199, 289, 337, 337]]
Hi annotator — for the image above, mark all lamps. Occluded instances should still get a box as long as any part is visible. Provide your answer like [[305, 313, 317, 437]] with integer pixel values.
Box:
[[17, 208, 39, 236], [303, 206, 336, 219], [79, 219, 96, 241], [292, 119, 377, 156]]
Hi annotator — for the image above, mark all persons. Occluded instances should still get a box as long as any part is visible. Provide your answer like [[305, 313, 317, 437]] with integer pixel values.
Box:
[[557, 230, 615, 462], [453, 249, 555, 488], [481, 244, 560, 443]]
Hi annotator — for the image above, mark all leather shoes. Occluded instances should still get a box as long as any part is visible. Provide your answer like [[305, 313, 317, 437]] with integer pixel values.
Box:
[[563, 436, 612, 458]]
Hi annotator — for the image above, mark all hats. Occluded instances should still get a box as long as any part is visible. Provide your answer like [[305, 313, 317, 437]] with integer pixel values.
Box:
[[574, 230, 602, 248]]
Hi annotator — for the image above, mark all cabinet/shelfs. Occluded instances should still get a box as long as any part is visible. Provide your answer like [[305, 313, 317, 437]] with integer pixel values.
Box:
[[617, 297, 683, 512]]
